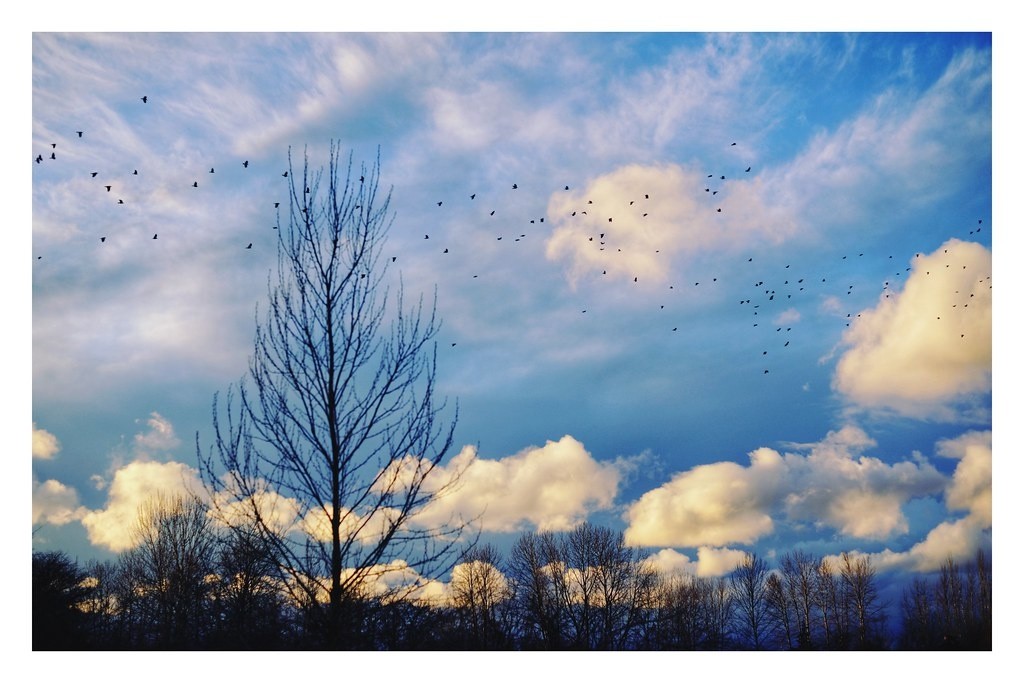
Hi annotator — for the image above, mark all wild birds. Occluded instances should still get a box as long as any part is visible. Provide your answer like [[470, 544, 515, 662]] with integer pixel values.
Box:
[[32, 95, 992, 374]]
